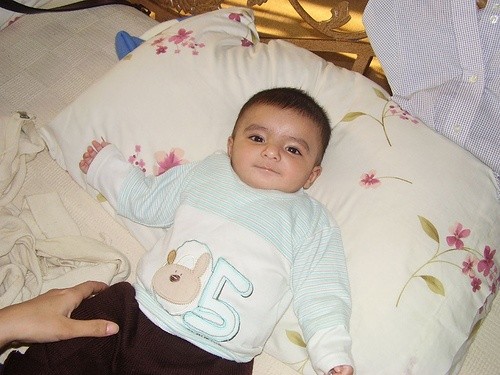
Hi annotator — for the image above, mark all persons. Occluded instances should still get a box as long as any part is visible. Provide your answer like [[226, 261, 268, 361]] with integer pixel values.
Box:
[[19, 78, 357, 375], [1, 278, 121, 357]]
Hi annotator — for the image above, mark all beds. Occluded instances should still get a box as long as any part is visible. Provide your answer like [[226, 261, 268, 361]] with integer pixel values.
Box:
[[2, 6, 500, 374]]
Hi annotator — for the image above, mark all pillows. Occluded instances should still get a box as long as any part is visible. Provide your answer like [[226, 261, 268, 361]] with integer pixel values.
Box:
[[39, 5, 497, 373]]
